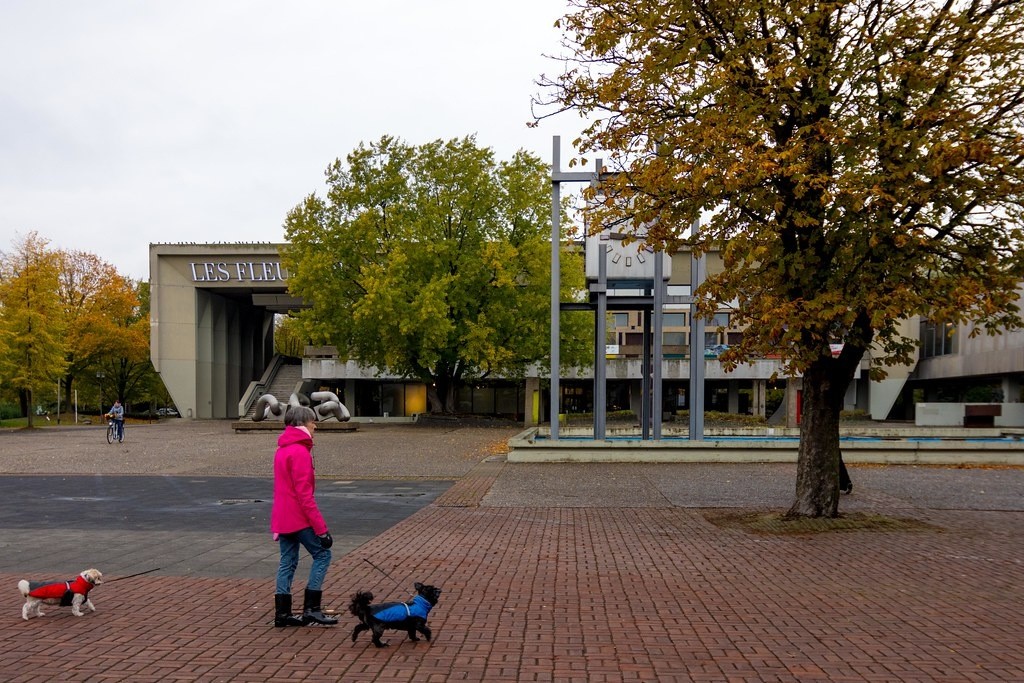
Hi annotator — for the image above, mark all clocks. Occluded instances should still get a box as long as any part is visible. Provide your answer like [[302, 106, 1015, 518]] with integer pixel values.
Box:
[[600, 210, 654, 266]]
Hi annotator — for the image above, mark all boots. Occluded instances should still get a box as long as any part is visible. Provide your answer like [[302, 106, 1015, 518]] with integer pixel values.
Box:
[[301, 589, 338, 626], [274, 593, 303, 627]]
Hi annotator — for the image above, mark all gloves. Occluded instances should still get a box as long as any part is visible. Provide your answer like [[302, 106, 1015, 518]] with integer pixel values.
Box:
[[319, 534, 333, 549]]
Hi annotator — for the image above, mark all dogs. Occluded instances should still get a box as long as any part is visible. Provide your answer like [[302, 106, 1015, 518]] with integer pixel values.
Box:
[[17, 567, 105, 623], [347, 581, 443, 650]]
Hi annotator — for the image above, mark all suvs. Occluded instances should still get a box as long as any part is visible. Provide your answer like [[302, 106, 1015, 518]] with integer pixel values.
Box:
[[158, 408, 178, 417]]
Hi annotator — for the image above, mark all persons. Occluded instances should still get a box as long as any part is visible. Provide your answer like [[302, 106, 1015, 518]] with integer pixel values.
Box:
[[108, 400, 124, 441], [270, 405, 339, 626]]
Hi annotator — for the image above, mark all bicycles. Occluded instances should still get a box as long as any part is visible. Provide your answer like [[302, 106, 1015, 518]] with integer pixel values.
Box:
[[104, 413, 125, 444]]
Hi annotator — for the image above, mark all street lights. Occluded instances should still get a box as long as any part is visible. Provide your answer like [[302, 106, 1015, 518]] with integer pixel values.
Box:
[[96, 371, 105, 424]]
